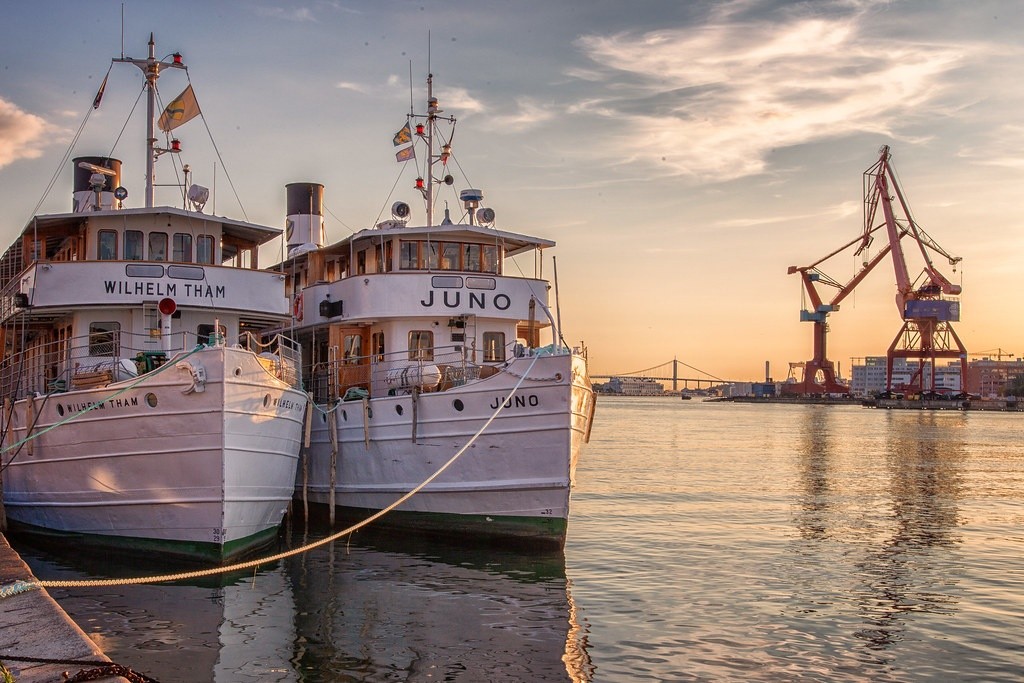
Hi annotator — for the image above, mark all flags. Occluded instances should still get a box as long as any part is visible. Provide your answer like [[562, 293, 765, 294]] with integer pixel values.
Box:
[[157, 85, 201, 133], [396, 146, 415, 162], [393, 121, 412, 146]]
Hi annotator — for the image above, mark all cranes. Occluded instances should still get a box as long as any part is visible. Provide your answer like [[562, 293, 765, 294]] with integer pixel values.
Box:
[[967, 347, 1014, 361], [786, 145, 969, 403]]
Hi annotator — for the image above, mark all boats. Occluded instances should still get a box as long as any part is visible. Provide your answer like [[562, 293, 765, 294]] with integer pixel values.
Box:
[[261, 30, 597, 553], [0, 0, 315, 570]]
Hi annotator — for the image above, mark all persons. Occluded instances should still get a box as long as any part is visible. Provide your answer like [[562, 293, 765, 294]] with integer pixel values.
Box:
[[154, 250, 164, 261], [101, 239, 112, 260]]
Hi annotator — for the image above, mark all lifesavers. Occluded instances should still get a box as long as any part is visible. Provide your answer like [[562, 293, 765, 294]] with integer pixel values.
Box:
[[293, 295, 304, 321]]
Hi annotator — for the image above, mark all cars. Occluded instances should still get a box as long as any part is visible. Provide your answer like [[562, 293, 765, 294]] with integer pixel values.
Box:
[[881, 390, 904, 399], [917, 392, 981, 400]]
